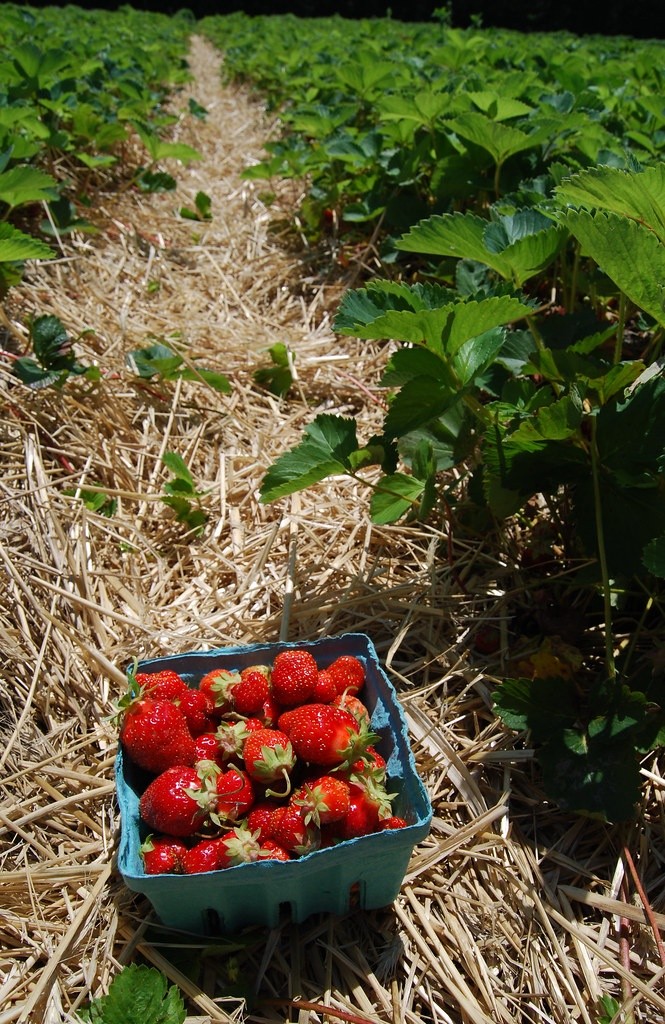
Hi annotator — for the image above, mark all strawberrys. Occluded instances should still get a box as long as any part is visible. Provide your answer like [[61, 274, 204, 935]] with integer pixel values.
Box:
[[109, 651, 407, 876]]
